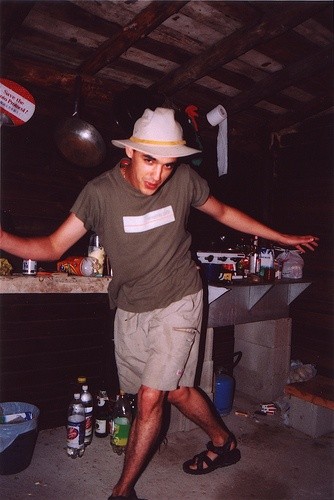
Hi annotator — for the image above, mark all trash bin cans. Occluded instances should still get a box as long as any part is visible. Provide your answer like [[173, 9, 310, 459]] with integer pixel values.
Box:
[[0, 401, 40, 475]]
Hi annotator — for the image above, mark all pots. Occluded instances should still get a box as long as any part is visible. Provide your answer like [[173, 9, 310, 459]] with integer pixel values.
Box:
[[49, 76, 107, 170]]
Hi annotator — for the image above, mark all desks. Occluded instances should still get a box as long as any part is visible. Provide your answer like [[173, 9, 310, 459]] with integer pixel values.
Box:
[[0, 275, 112, 294]]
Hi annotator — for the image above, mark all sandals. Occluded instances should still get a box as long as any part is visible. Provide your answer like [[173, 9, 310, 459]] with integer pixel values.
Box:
[[108, 489, 137, 500], [183, 432, 241, 474]]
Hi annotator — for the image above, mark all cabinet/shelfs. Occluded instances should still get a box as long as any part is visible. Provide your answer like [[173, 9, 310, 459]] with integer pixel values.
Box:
[[166, 278, 312, 432]]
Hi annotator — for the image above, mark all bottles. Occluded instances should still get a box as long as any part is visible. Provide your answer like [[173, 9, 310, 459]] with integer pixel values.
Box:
[[88, 233, 105, 277], [250, 236, 260, 273], [80, 385, 93, 445], [76, 376, 87, 394], [56, 255, 101, 276], [65, 393, 85, 459], [94, 387, 137, 455]]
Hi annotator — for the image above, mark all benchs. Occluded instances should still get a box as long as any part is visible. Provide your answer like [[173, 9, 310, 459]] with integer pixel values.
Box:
[[284, 377, 334, 438]]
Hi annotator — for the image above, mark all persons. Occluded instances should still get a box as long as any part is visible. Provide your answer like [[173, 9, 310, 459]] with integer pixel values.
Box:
[[0, 107, 320, 500]]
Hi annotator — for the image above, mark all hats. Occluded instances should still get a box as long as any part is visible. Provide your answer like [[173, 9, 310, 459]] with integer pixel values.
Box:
[[111, 107, 202, 159]]
